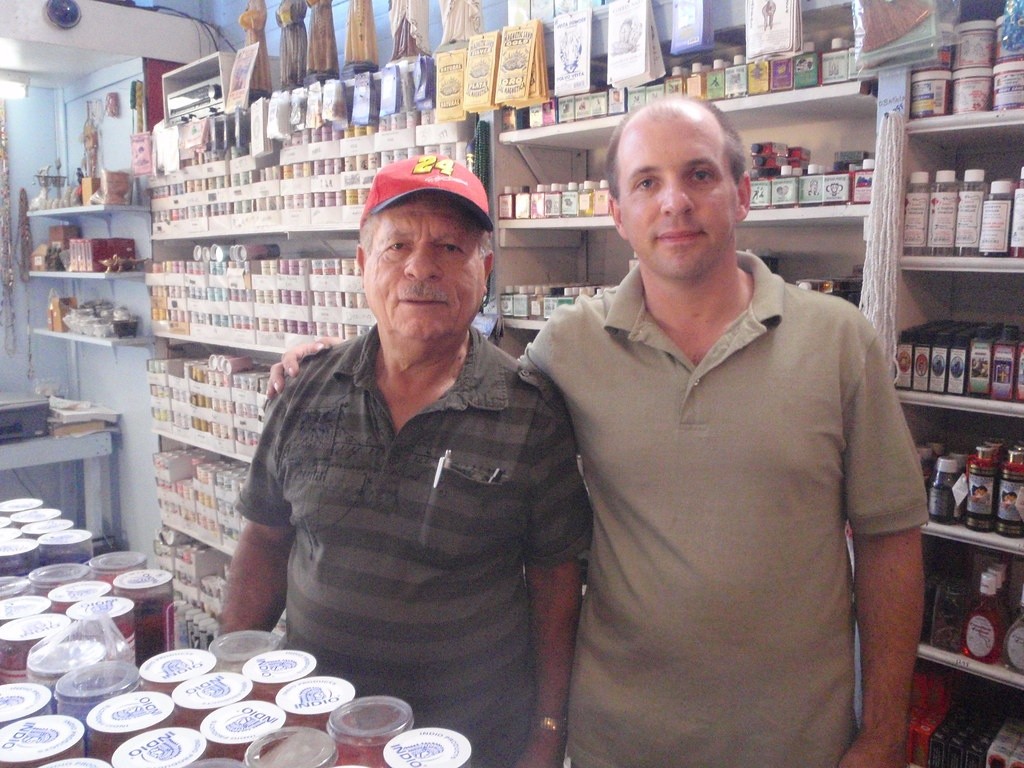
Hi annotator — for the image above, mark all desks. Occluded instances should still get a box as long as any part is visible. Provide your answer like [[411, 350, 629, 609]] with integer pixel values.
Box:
[[1, 432, 112, 540]]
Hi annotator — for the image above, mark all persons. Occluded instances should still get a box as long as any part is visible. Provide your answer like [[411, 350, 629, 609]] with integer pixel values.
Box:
[[972, 485, 987, 496], [268, 92, 931, 768], [238, 0, 484, 94], [215, 152, 594, 768], [1002, 491, 1017, 502]]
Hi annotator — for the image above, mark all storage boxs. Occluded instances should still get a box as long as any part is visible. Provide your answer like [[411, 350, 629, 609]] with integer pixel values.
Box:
[[0, 391, 49, 440]]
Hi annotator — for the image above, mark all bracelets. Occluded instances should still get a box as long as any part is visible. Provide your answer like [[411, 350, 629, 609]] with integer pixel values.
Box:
[[537, 716, 566, 732]]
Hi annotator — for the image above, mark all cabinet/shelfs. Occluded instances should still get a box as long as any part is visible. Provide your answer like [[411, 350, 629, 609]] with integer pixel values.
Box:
[[144, 41, 1024, 767], [27, 206, 151, 348]]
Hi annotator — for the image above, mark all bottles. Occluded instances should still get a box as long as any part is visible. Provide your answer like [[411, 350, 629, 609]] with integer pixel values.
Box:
[[795, 278, 834, 294], [500, 37, 857, 135], [910, 434, 1024, 538], [748, 140, 875, 211], [910, 16, 1024, 117], [0, 495, 475, 768], [894, 321, 1024, 400], [902, 167, 1024, 258], [627, 250, 639, 272], [498, 179, 610, 219], [499, 281, 619, 322], [926, 554, 1024, 671]]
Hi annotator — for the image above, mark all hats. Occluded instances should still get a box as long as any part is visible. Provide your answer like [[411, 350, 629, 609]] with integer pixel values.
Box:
[[360, 153, 493, 232]]
